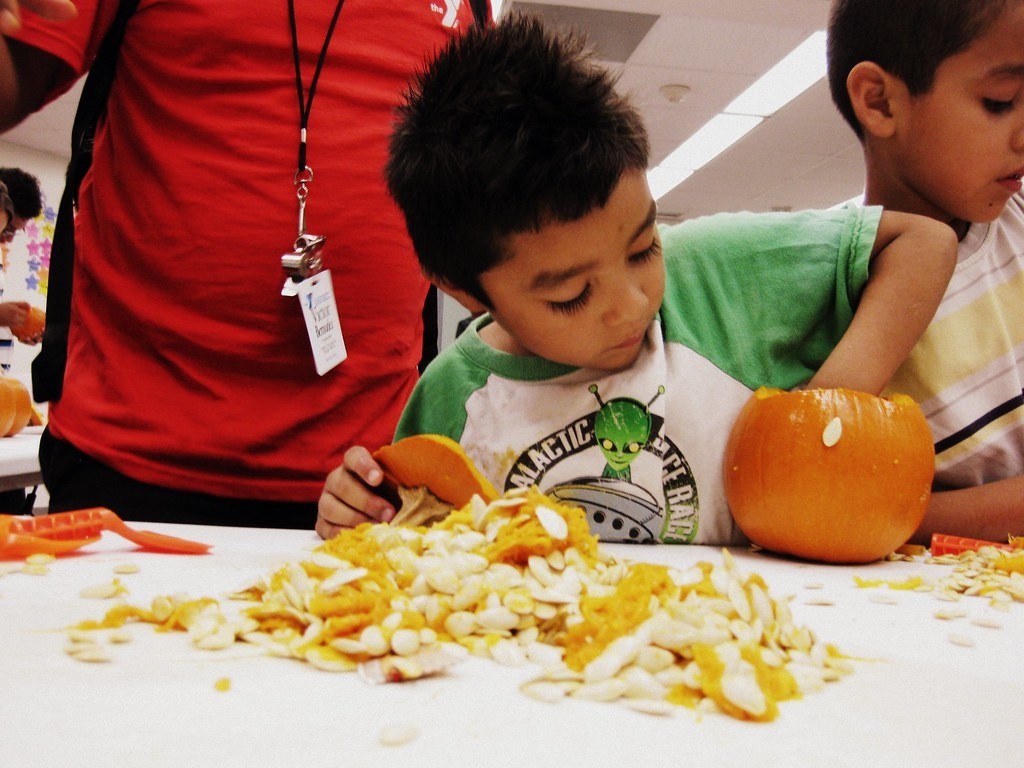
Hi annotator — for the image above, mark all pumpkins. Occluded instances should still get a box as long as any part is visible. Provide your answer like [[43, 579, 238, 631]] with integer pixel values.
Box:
[[9, 308, 46, 340], [0, 370, 32, 437]]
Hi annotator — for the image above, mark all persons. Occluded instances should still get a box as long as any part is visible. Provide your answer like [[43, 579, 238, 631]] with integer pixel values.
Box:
[[0, 0, 500, 524], [822, 0, 1024, 541], [317, 16, 959, 541]]
